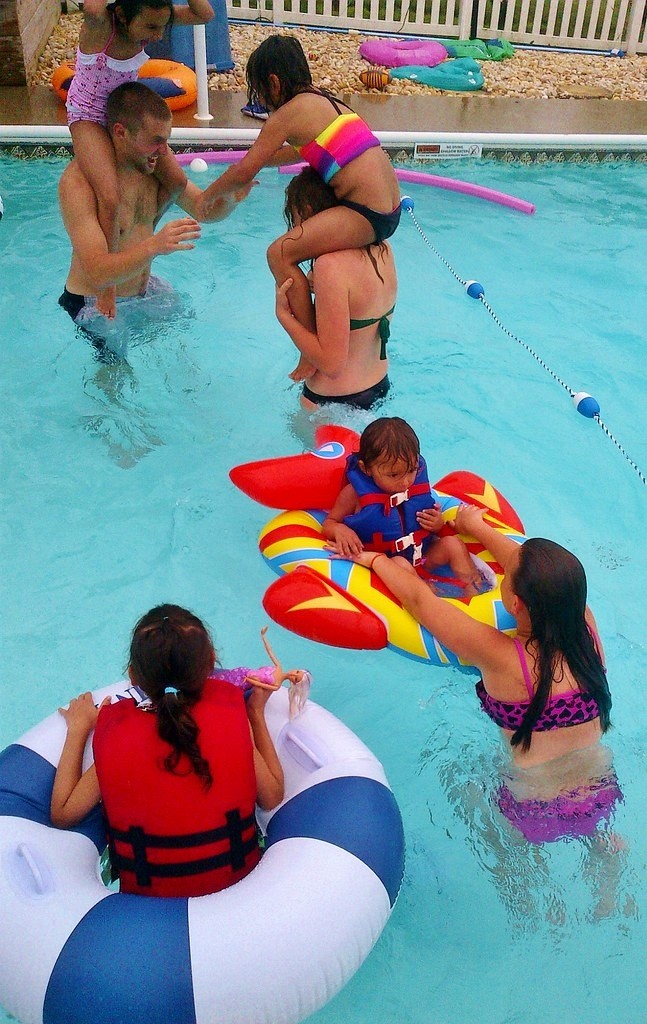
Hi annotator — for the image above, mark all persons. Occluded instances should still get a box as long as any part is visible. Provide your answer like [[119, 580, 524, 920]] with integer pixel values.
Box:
[[187, 33, 404, 382], [66, 0, 215, 321], [207, 625, 312, 692], [322, 415, 485, 599], [273, 165, 397, 414], [51, 600, 284, 900], [54, 82, 260, 323], [323, 501, 626, 846]]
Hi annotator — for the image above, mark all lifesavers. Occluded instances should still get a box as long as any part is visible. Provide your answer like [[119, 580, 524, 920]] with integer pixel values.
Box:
[[357, 39, 486, 92], [1, 664, 407, 1023], [51, 55, 198, 113], [171, 147, 251, 167], [276, 161, 536, 216]]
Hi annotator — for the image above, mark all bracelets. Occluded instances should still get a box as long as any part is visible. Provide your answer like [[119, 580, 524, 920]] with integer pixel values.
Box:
[[371, 554, 383, 569]]
